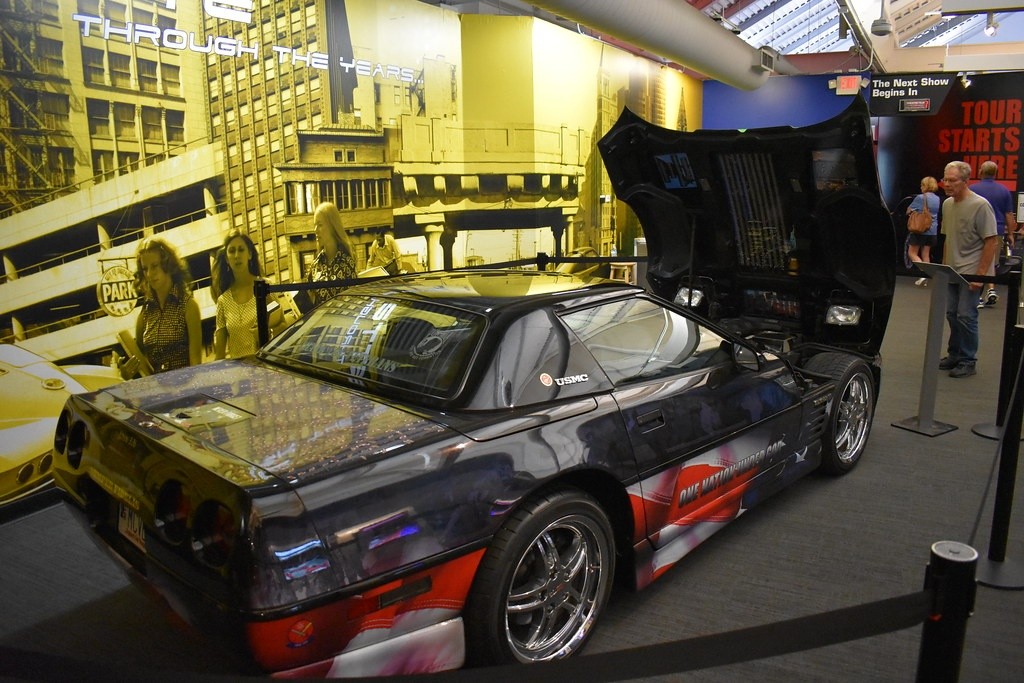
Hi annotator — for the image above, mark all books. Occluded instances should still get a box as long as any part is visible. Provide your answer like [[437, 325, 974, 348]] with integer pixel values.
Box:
[[267, 301, 281, 327], [284, 289, 314, 317], [116, 330, 154, 376]]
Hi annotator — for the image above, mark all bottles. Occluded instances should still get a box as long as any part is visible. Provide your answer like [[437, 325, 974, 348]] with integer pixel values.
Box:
[[610, 243, 617, 257]]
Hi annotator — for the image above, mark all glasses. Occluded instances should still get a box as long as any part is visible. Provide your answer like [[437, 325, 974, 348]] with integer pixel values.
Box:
[[941, 177, 966, 183]]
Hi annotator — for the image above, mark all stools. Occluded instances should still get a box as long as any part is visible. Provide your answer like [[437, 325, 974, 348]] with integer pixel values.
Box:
[[609, 261, 637, 286]]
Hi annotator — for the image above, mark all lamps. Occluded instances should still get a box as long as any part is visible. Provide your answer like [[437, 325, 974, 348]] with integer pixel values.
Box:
[[984, 12, 1000, 38], [961, 71, 971, 88]]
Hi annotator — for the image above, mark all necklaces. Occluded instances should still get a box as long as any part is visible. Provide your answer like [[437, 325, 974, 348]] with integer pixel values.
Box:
[[236, 276, 252, 323]]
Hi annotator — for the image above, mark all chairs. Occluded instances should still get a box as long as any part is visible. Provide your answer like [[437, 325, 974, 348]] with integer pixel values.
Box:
[[366, 316, 445, 387], [995, 232, 1022, 287]]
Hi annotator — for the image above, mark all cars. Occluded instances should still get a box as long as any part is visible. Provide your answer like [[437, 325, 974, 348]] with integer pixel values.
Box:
[[51, 89, 902, 682]]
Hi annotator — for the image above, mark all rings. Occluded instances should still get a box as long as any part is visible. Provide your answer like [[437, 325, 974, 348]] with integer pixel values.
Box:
[[131, 371, 134, 373]]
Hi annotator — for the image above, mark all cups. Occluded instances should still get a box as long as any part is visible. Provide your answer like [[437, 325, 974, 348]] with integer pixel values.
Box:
[[1005, 239, 1012, 257]]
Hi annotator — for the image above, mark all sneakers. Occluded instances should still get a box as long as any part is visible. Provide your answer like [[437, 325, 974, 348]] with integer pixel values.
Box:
[[950, 361, 975, 378], [985, 290, 998, 305], [978, 298, 984, 308], [938, 356, 954, 370]]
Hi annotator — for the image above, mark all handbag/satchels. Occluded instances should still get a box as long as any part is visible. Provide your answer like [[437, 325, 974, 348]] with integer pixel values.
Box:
[[907, 194, 932, 234]]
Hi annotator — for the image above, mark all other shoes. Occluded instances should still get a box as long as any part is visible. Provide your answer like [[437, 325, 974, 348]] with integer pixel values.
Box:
[[915, 276, 927, 287]]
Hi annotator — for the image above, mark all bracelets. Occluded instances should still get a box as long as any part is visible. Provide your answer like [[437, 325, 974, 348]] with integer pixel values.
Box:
[[270, 329, 273, 339]]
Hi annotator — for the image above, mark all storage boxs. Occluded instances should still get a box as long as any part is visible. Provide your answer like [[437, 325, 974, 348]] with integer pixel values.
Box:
[[634, 238, 648, 256]]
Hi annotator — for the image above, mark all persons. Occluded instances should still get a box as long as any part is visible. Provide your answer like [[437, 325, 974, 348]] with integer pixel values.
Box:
[[117, 236, 202, 381], [292, 202, 358, 319], [939, 162, 998, 379], [970, 162, 1015, 308], [906, 177, 940, 286], [366, 226, 402, 276], [209, 228, 288, 360]]
